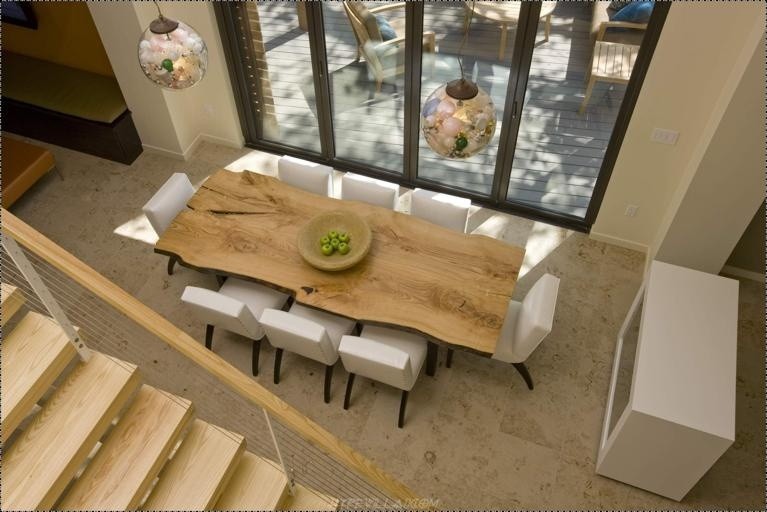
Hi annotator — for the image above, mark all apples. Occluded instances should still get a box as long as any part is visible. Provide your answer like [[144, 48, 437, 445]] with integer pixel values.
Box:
[[338, 233, 349, 243], [320, 243, 333, 255], [330, 239, 340, 249], [320, 236, 330, 244], [338, 243, 348, 254], [328, 231, 338, 239]]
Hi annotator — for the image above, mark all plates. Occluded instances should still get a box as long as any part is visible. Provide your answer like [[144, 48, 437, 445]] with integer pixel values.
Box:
[[295, 209, 372, 272]]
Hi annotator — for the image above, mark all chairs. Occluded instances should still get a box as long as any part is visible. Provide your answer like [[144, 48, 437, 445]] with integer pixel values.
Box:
[[277, 154, 471, 234], [142, 173, 195, 277], [342, 0, 438, 97], [576, 0, 654, 114], [176, 274, 432, 428], [492, 272, 562, 391]]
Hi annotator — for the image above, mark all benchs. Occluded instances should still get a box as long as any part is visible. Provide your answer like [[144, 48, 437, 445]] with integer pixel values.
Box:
[[1, 51, 130, 164], [1, 136, 66, 210]]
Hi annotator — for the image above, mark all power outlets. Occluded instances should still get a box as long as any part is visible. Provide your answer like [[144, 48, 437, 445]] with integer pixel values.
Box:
[[625, 204, 639, 219]]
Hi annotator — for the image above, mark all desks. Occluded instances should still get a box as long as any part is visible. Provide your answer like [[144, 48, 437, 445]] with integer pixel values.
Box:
[[455, 0, 567, 63]]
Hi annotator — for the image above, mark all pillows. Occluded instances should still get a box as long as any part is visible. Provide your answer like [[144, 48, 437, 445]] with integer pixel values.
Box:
[[610, 0, 655, 24]]
